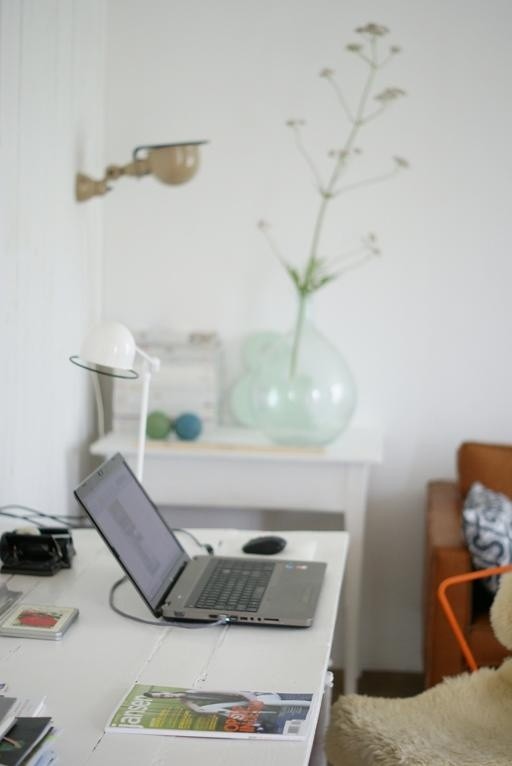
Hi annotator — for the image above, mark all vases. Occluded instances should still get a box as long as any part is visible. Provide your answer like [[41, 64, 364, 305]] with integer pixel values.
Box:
[[230, 323, 359, 449]]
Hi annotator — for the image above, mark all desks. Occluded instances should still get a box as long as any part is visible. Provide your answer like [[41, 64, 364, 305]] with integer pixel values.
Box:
[[85, 419, 382, 695], [0, 523, 351, 765]]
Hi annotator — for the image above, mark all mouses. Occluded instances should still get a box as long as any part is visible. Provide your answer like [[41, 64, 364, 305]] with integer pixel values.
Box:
[[242, 535, 287, 555]]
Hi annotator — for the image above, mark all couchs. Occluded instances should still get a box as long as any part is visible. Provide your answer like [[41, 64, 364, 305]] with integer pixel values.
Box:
[[422, 441, 512, 690]]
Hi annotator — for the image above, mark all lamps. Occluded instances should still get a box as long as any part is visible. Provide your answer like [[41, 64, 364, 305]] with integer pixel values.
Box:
[[68, 321, 160, 482]]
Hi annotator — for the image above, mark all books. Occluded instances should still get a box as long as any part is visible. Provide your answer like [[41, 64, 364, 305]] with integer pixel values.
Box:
[[104, 680, 319, 743], [0, 683, 64, 766]]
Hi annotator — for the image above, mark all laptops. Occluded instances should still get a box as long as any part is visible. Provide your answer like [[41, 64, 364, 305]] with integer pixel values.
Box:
[[71, 450, 328, 627]]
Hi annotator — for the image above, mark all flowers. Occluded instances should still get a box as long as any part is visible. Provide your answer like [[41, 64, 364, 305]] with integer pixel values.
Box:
[[256, 24, 408, 382]]
